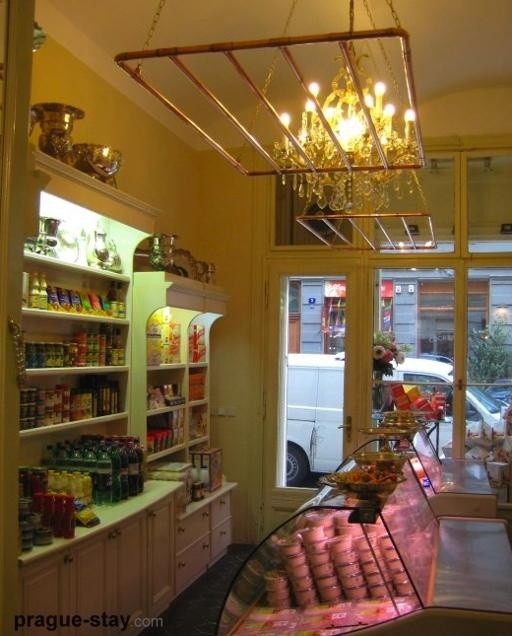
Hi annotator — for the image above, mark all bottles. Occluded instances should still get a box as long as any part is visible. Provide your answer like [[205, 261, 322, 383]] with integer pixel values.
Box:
[[29, 271, 48, 311], [29, 434, 144, 539], [106, 281, 126, 319], [190, 464, 209, 499]]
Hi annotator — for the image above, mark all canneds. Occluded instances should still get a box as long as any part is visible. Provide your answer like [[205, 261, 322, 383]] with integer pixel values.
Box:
[[24, 322, 125, 368], [111, 301, 126, 319], [20, 383, 118, 431], [18, 466, 53, 550], [192, 480, 205, 501]]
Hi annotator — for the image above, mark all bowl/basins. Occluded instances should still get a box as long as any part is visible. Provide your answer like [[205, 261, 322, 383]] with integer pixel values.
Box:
[[351, 451, 409, 473]]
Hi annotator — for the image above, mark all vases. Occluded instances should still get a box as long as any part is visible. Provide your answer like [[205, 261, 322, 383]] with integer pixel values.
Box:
[[149, 231, 178, 272], [371, 371, 383, 410]]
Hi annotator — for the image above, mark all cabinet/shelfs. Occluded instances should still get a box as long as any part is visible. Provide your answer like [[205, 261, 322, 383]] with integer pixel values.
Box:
[[131, 270, 238, 594], [0, 150, 184, 636]]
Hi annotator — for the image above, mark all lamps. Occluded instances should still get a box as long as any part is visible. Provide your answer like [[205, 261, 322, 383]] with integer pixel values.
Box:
[[270, 0, 421, 212]]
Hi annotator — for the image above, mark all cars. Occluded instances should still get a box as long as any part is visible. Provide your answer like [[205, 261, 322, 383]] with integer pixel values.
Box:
[[479, 376, 512, 407]]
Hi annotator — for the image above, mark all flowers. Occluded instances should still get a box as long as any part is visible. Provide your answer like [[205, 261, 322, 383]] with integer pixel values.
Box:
[[371, 328, 415, 409]]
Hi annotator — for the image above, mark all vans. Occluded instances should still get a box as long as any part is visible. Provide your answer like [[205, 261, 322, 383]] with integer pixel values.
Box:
[[281, 352, 511, 489]]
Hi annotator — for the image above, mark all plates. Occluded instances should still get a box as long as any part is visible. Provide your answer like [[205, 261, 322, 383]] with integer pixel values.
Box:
[[318, 470, 408, 501]]
